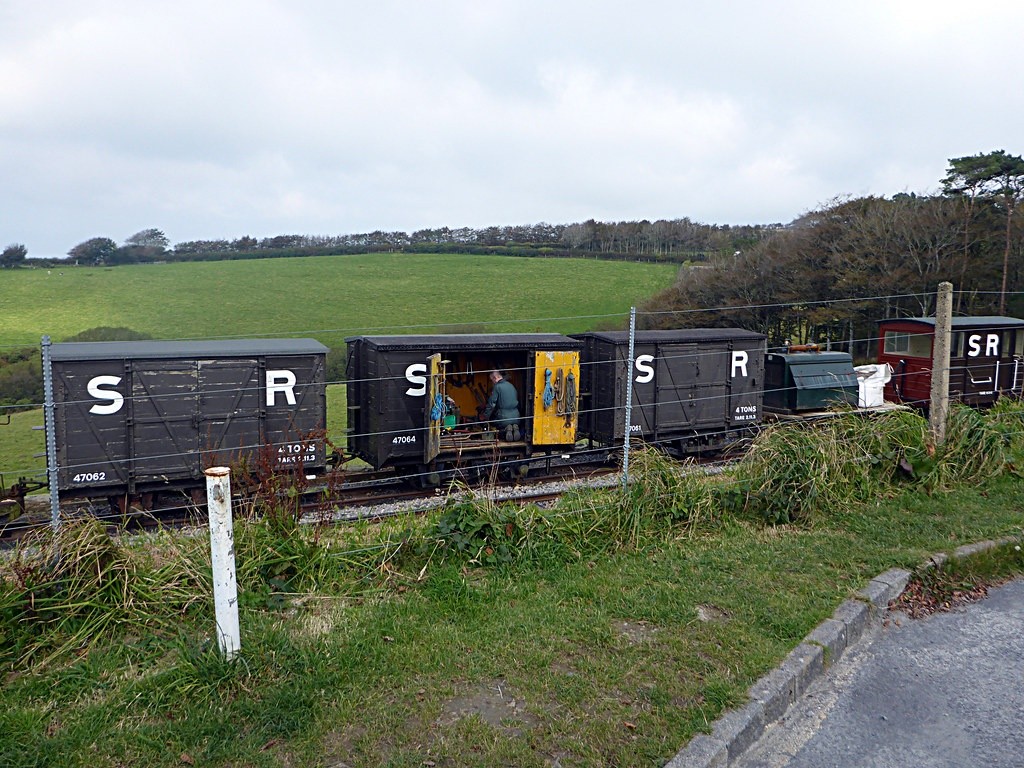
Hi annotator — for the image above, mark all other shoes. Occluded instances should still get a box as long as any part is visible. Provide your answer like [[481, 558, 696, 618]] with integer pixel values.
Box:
[[506, 424, 513, 442], [512, 424, 520, 442]]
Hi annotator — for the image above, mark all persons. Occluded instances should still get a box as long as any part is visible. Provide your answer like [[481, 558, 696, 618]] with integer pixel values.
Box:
[[480, 371, 520, 440]]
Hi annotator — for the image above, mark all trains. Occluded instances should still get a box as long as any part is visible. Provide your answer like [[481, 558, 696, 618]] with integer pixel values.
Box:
[[0, 316, 1024, 528]]
